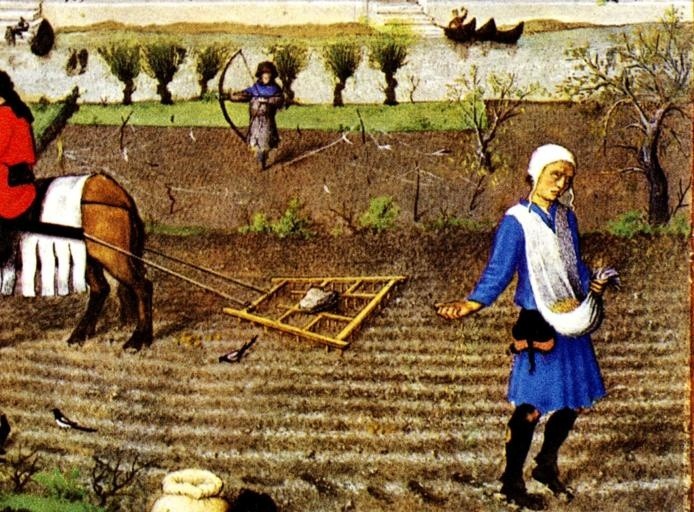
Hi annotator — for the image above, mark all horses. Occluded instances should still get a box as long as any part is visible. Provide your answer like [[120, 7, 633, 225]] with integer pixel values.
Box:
[[0, 172, 154, 353]]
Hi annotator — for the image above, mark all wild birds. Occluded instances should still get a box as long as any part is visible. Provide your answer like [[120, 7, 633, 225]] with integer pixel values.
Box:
[[49, 408, 100, 434], [218, 334, 260, 364]]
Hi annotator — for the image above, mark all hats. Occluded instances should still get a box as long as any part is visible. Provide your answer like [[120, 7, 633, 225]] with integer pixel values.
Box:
[[527, 142, 577, 184], [255, 61, 278, 74]]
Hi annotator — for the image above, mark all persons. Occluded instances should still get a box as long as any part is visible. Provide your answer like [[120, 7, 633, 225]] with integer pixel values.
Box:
[[0, 69, 37, 271], [433, 145, 608, 508], [228, 60, 284, 145]]
